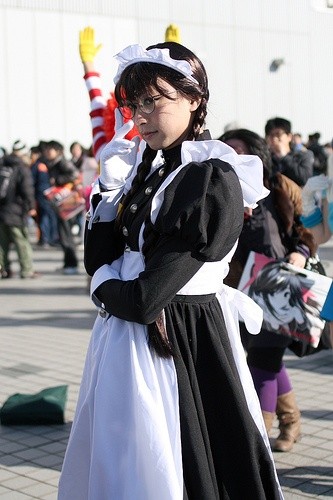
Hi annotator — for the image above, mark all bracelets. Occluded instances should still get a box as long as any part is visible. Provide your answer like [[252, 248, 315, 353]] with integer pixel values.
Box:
[[296, 245, 310, 258]]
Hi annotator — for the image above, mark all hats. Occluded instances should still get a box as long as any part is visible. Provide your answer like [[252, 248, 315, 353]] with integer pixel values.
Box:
[[12, 140, 27, 151]]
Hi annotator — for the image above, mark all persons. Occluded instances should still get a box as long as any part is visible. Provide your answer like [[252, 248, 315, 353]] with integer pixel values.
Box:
[[219, 129, 317, 452], [57, 42, 284, 500], [264, 118, 333, 349], [0, 140, 100, 278]]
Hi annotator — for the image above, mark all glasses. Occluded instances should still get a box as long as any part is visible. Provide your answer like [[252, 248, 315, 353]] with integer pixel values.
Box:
[[118, 90, 180, 119]]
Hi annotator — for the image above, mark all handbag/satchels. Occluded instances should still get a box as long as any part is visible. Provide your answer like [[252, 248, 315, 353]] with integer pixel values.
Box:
[[237, 251, 333, 348]]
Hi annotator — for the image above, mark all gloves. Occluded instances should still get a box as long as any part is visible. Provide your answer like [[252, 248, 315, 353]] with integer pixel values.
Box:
[[100, 108, 140, 190], [111, 255, 124, 276]]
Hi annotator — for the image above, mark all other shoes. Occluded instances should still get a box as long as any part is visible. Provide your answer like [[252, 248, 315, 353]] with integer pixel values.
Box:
[[21, 271, 44, 279], [1, 270, 17, 278], [59, 267, 81, 275]]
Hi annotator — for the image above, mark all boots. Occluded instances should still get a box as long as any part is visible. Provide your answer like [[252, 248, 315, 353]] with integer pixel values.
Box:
[[275, 390, 303, 452], [261, 411, 278, 434]]
